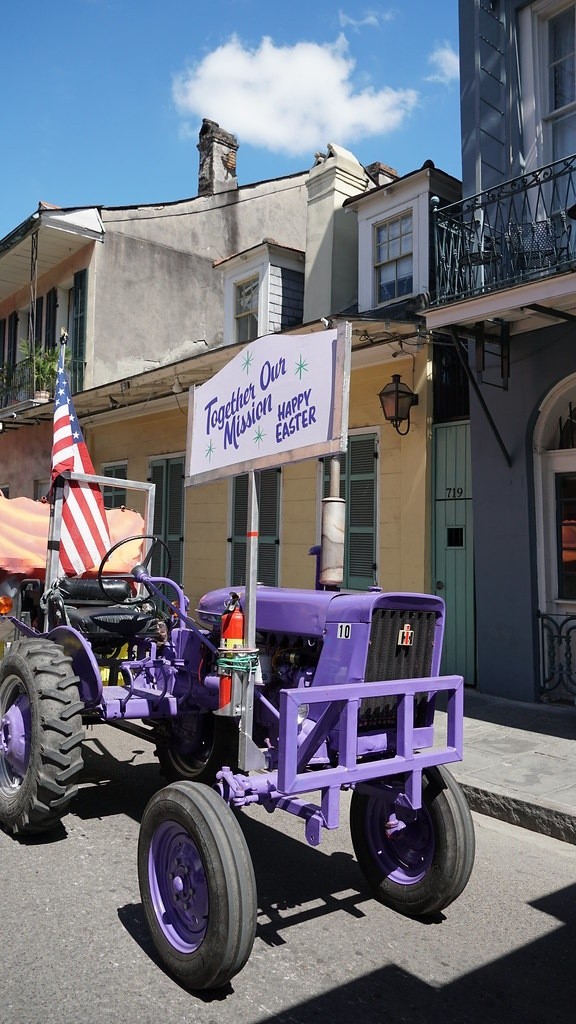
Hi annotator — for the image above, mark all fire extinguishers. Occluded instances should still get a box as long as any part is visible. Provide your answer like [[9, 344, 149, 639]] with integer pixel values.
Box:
[[217, 590, 246, 712]]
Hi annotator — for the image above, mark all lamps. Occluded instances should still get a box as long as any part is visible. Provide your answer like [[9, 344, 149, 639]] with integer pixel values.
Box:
[[172, 376, 184, 393], [108, 395, 119, 408], [377, 374, 419, 436]]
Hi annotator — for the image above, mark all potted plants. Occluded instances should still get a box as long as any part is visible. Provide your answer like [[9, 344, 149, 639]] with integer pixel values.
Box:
[[16, 337, 72, 403], [0, 361, 27, 405]]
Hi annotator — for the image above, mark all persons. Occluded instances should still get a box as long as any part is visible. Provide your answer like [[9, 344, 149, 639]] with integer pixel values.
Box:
[[0, 569, 43, 630]]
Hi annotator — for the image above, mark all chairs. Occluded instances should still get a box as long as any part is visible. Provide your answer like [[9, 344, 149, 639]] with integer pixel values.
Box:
[[57, 579, 155, 638], [505, 220, 572, 283]]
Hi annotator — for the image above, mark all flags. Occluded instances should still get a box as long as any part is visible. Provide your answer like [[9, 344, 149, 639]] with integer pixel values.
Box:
[[51, 356, 110, 582]]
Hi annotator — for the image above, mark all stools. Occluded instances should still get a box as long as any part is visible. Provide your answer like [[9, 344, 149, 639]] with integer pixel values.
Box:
[[459, 251, 504, 294]]
